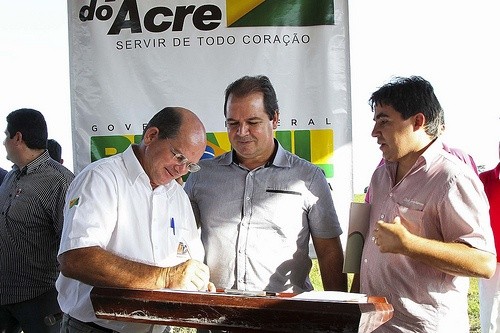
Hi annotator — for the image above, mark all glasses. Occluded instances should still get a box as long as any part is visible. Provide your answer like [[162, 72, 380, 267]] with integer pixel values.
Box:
[[158, 130, 202, 173]]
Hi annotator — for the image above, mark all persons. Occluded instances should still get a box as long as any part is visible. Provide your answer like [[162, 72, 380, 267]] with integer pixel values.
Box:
[[353, 75, 497, 333], [183, 75, 348, 292], [54, 106, 217, 333], [479, 140, 500, 333], [0, 108, 77, 333]]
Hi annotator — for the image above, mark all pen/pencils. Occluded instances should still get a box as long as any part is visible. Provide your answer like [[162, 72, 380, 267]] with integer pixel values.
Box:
[[185, 243, 207, 292], [169, 217, 175, 235], [226, 290, 282, 296]]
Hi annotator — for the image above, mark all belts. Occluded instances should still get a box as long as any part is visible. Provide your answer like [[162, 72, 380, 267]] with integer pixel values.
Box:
[[62, 314, 114, 333]]
